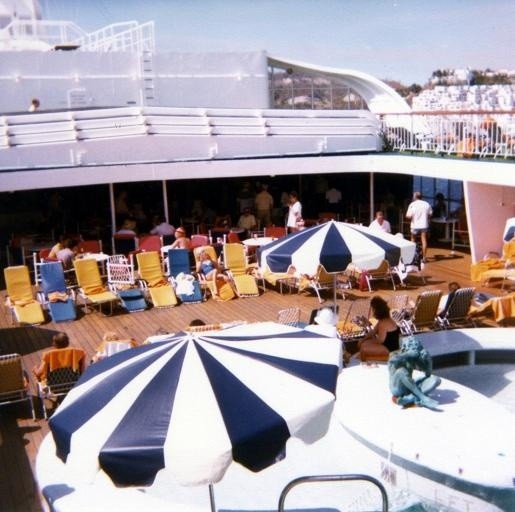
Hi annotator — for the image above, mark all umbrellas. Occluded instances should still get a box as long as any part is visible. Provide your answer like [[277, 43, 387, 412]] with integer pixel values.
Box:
[[46, 321, 346, 511], [259, 218, 416, 317]]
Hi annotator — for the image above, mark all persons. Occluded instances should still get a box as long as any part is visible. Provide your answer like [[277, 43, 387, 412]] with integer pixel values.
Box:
[[437, 284, 463, 316], [32, 329, 70, 377], [103, 332, 122, 344], [389, 335, 441, 407], [356, 294, 399, 363], [189, 319, 207, 326], [3, 180, 449, 309], [154, 327, 166, 336], [28, 99, 40, 112], [380, 93, 515, 158]]
